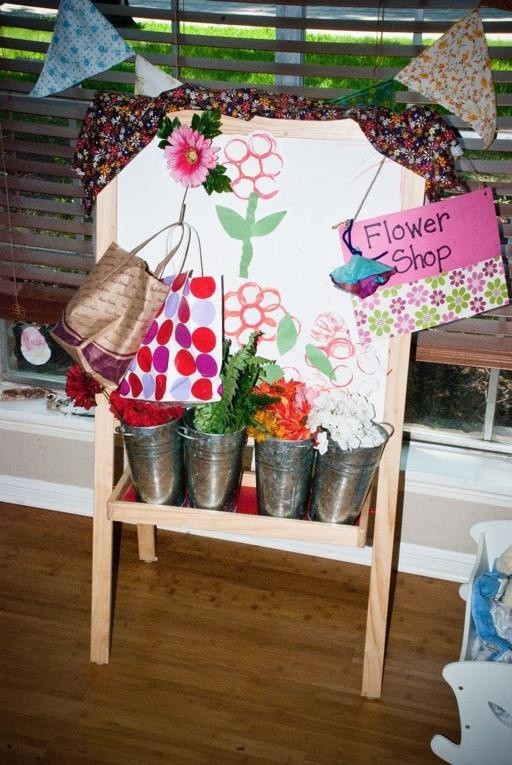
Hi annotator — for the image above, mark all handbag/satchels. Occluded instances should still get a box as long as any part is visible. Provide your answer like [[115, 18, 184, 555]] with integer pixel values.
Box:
[[49, 222, 225, 405], [338, 101, 510, 347]]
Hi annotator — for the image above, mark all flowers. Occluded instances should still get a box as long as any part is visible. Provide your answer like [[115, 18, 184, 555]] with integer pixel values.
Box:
[[65, 365, 186, 426], [248, 377, 384, 456], [156, 107, 233, 195]]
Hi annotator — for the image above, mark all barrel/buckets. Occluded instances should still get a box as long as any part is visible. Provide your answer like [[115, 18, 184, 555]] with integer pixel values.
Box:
[[177, 422, 247, 512]]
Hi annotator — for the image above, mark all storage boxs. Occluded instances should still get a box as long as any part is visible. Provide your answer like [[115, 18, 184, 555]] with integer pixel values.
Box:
[[430, 520, 512, 764]]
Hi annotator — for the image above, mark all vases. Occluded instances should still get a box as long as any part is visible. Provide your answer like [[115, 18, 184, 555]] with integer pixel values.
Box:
[[174, 407, 248, 512]]
